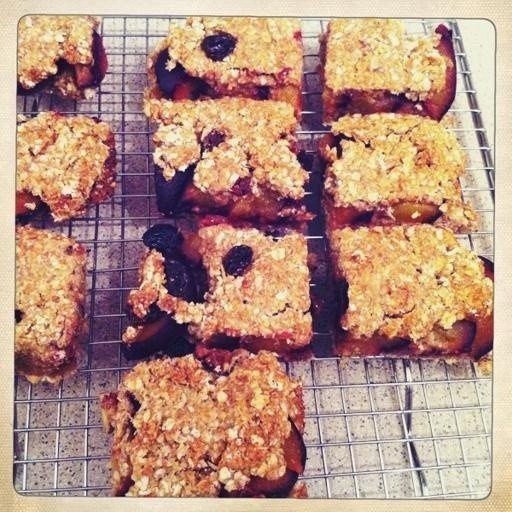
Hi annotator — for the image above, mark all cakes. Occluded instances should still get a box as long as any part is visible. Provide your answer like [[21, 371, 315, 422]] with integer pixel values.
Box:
[[17, 14, 109, 101], [316, 18, 457, 126], [142, 96, 318, 225], [16, 111, 117, 221], [142, 16, 303, 124], [318, 111, 481, 233], [15, 223, 89, 385], [122, 212, 324, 359], [100, 349, 307, 498], [325, 225, 493, 360]]
